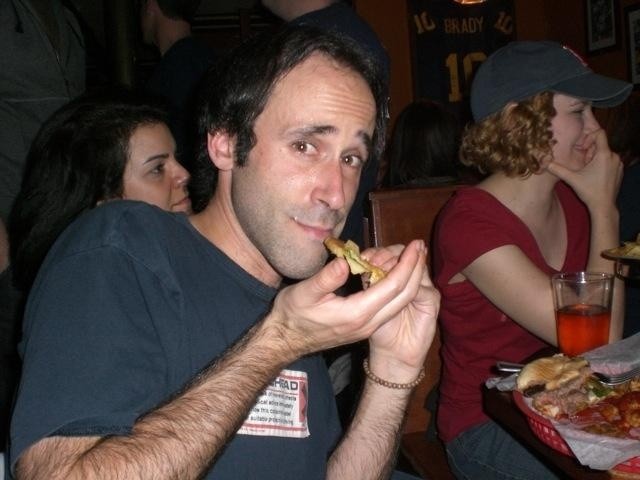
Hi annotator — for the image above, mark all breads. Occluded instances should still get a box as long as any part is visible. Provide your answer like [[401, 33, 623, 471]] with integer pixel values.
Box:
[[515, 356, 580, 390]]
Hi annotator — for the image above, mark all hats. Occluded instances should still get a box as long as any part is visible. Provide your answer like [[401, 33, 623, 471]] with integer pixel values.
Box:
[[470, 41, 633, 120]]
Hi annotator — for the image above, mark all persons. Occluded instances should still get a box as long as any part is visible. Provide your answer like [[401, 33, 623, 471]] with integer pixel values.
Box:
[[431, 40, 625, 480]]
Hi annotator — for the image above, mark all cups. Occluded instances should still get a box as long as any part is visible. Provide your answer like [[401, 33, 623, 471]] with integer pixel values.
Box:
[[550, 270, 615, 358]]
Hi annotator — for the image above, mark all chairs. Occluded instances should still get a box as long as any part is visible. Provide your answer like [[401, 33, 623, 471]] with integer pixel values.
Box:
[[356, 180, 481, 476]]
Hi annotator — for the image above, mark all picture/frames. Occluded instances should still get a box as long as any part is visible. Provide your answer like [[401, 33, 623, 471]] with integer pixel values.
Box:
[[581, 1, 640, 89]]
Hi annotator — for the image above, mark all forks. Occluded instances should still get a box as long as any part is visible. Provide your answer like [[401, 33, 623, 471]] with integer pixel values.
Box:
[[495, 359, 640, 385]]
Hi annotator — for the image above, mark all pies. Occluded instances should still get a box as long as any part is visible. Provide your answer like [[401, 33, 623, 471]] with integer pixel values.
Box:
[[322, 236, 388, 288]]
